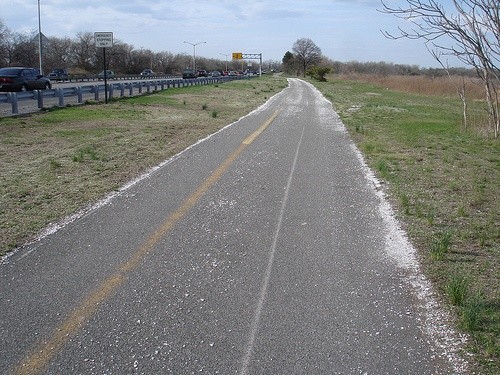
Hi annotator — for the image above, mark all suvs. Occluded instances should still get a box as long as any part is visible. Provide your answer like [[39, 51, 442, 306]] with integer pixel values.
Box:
[[50, 68, 68, 82]]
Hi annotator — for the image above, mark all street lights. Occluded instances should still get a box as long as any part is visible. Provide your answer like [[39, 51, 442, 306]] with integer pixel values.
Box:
[[184, 41, 206, 73], [219, 53, 231, 71]]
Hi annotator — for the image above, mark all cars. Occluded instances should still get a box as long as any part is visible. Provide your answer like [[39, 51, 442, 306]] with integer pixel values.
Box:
[[140, 69, 153, 79], [182, 70, 196, 79], [98, 70, 114, 80], [0, 67, 52, 93], [198, 70, 208, 77], [207, 70, 266, 77]]
